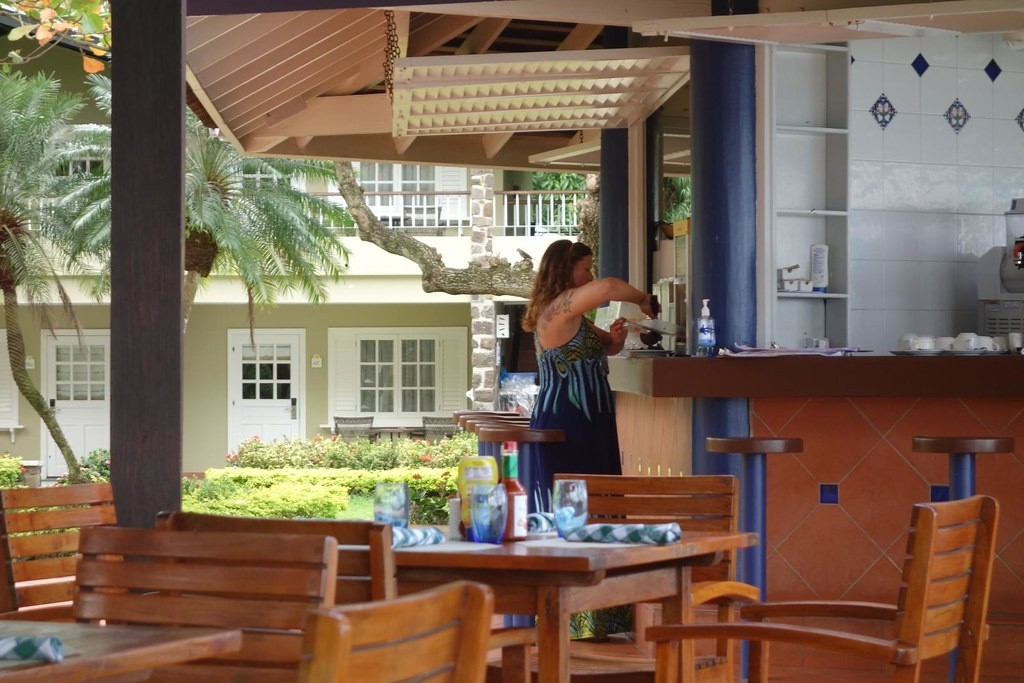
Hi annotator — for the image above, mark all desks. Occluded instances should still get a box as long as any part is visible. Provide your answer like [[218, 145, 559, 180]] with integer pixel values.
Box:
[[320, 425, 461, 441]]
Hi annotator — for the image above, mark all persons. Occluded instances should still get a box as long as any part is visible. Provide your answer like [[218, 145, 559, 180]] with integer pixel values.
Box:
[[503, 239, 664, 644]]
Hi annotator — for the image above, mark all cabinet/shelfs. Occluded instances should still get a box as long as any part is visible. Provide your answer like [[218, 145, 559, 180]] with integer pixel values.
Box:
[[770, 42, 852, 349]]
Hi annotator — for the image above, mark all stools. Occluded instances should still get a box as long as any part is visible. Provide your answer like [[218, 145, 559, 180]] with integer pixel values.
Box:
[[453, 410, 566, 647], [703, 435, 804, 680], [912, 434, 1017, 680]]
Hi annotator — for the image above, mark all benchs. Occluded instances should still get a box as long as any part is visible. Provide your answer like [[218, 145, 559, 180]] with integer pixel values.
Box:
[[155, 510, 538, 683], [0, 482, 118, 623], [73, 523, 339, 683]]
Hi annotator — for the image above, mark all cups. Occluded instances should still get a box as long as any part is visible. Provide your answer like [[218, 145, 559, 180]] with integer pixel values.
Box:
[[372, 482, 409, 527], [903, 332, 1021, 353], [551, 477, 588, 538], [470, 479, 509, 545]]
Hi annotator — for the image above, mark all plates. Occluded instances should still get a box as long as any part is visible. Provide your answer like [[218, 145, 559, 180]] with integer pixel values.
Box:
[[889, 347, 1007, 357], [627, 348, 672, 354], [628, 320, 677, 337]]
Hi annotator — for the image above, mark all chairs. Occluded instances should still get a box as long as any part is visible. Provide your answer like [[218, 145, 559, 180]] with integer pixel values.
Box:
[[422, 414, 460, 441], [641, 492, 999, 681], [487, 470, 762, 683], [295, 579, 495, 681], [331, 416, 378, 443]]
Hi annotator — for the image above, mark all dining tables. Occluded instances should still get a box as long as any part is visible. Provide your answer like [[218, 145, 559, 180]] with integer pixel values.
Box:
[[0, 618, 245, 682], [339, 521, 761, 683]]
